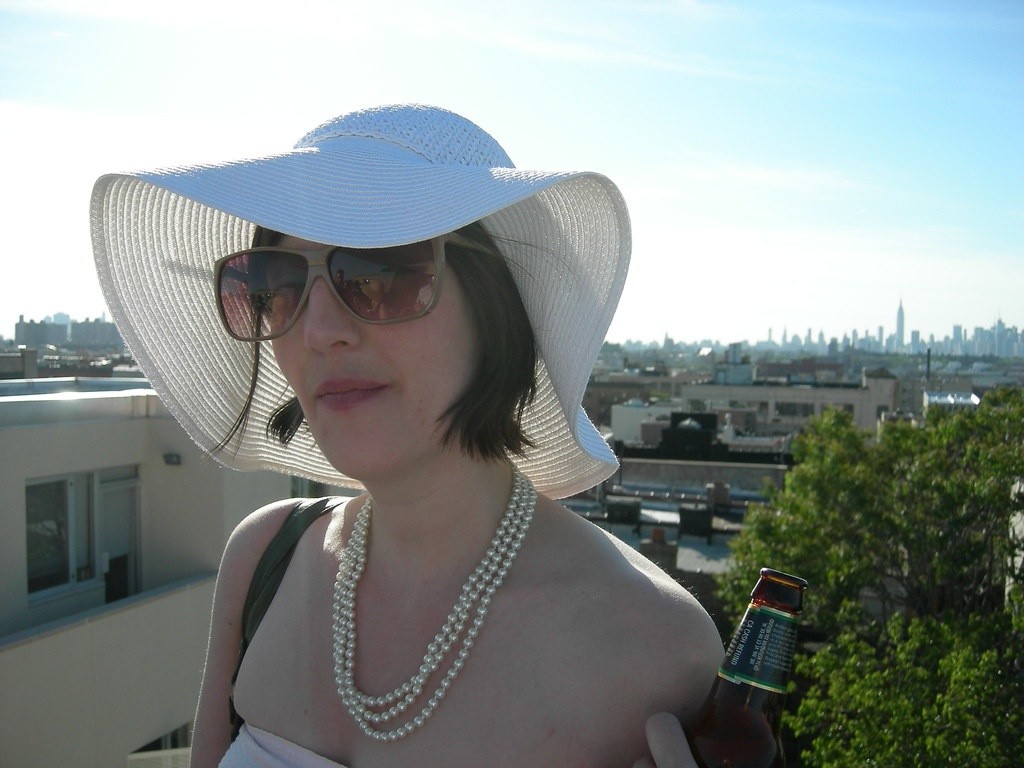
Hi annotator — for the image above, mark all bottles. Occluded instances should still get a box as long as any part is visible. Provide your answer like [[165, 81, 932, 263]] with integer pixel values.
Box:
[[681, 567, 808, 768]]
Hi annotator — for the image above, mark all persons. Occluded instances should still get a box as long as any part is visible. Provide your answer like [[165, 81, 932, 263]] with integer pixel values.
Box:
[[90, 104, 775, 768]]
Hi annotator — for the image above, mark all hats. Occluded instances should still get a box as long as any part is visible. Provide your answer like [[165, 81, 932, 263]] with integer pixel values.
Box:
[[90, 103, 633, 502]]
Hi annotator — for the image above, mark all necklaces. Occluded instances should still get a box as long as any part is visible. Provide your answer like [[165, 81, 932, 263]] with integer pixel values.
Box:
[[333, 460, 537, 741]]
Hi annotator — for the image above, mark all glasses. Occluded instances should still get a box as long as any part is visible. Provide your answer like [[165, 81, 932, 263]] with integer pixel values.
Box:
[[213, 232, 497, 341]]
